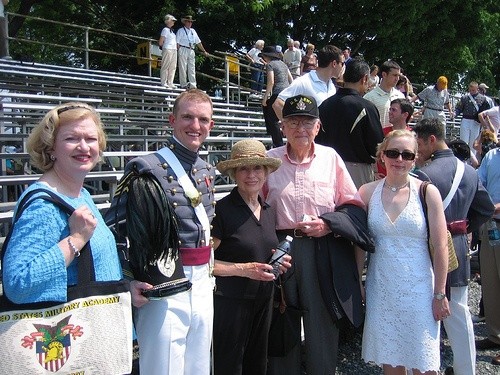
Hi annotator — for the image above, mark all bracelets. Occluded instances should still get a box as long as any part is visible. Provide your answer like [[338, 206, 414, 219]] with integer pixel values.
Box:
[[67, 236, 80, 257]]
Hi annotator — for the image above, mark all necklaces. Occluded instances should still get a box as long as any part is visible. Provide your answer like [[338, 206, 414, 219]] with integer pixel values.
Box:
[[384, 181, 410, 191]]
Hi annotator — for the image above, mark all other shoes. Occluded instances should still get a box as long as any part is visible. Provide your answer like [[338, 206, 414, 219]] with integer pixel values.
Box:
[[161, 82, 264, 99]]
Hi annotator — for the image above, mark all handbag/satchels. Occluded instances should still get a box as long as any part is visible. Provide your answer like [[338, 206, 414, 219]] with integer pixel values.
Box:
[[0, 188, 132, 375]]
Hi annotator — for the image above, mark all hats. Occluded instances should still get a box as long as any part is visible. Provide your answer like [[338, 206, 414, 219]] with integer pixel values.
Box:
[[180, 15, 197, 24], [479, 84, 490, 88], [257, 45, 282, 59], [282, 95, 319, 118], [164, 15, 177, 23], [219, 140, 283, 177], [127, 165, 192, 299], [438, 76, 448, 89], [449, 139, 471, 162]]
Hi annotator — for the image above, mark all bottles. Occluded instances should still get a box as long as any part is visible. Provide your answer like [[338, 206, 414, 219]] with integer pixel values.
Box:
[[487, 217, 499, 246], [265, 234, 293, 280]]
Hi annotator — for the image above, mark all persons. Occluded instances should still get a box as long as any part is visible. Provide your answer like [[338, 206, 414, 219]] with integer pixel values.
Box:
[[408, 75, 454, 137], [159, 14, 178, 89], [412, 117, 495, 375], [0, 0, 13, 60], [478, 104, 500, 135], [256, 45, 293, 148], [479, 83, 494, 107], [246, 39, 266, 99], [448, 139, 479, 169], [473, 113, 497, 161], [374, 97, 418, 181], [317, 60, 384, 190], [351, 130, 451, 375], [474, 147, 500, 366], [453, 82, 491, 164], [104, 88, 216, 375], [257, 94, 367, 375], [275, 38, 415, 98], [175, 16, 209, 90], [212, 139, 292, 374], [1, 101, 137, 339], [361, 59, 406, 128]]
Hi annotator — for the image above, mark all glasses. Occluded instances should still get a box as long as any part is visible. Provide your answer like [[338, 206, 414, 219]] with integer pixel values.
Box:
[[381, 150, 416, 161], [287, 45, 344, 67], [285, 118, 317, 128], [170, 20, 176, 21], [185, 21, 192, 23]]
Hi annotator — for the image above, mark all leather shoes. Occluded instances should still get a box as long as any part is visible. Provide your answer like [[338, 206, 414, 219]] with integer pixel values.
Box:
[[493, 355, 500, 365], [475, 339, 499, 348]]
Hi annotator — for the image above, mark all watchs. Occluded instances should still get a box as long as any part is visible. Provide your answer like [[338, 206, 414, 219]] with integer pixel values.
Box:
[[434, 292, 446, 300]]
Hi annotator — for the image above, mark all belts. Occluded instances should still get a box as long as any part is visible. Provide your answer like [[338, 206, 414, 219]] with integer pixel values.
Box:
[[276, 228, 342, 238]]
